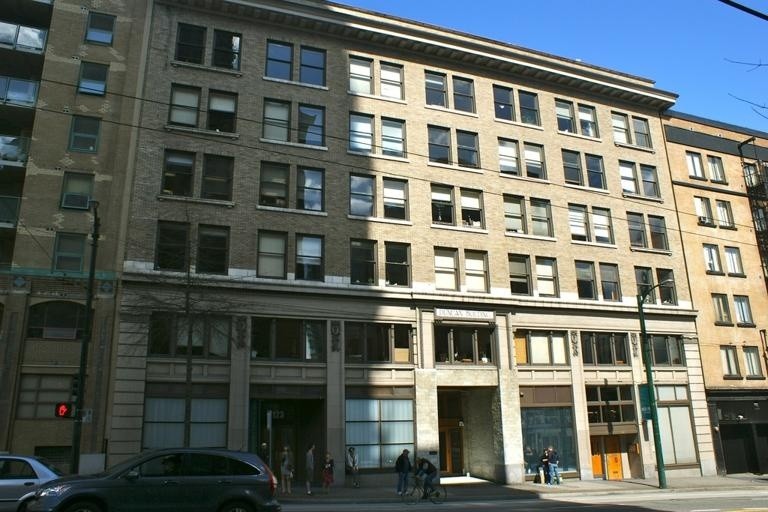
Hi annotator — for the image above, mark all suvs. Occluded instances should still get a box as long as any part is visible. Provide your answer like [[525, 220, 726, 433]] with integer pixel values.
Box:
[[17, 445, 282, 511]]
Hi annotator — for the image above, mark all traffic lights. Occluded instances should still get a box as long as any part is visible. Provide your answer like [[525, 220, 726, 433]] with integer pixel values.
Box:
[[55, 401, 73, 419]]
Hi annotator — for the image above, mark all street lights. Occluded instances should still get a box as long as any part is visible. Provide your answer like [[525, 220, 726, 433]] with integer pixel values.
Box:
[[68, 198, 103, 481], [635, 276, 679, 490]]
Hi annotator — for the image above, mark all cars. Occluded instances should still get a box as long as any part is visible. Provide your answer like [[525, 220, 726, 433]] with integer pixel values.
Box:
[[2, 452, 62, 510]]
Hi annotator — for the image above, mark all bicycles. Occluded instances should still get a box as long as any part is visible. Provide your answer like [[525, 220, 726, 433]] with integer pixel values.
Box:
[[403, 473, 447, 507]]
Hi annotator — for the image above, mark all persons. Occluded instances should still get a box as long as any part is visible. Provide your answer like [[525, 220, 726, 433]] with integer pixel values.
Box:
[[279, 444, 296, 495], [349, 447, 360, 488], [321, 452, 334, 494], [524, 445, 537, 474], [541, 445, 560, 485], [305, 441, 315, 495], [414, 457, 438, 499], [260, 441, 270, 467], [395, 449, 412, 495]]
